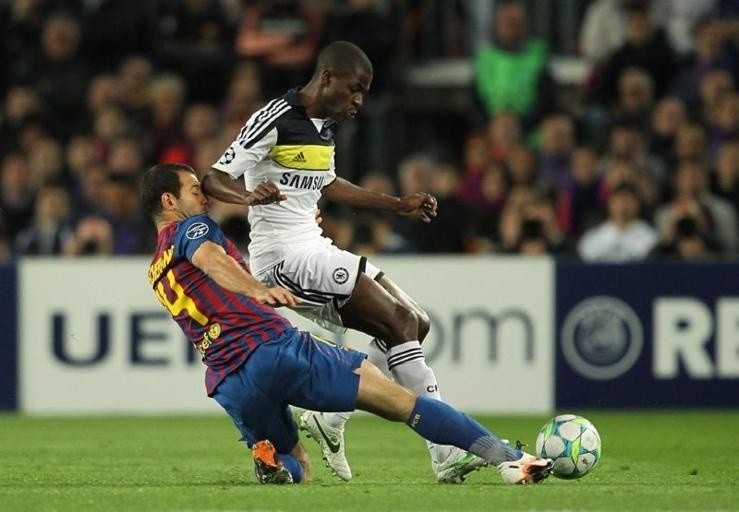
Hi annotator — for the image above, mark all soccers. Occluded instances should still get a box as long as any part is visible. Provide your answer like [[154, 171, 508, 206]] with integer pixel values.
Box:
[[536, 414, 601, 479]]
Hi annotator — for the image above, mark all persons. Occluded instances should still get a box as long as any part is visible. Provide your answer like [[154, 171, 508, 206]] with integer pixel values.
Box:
[[201, 39, 511, 485], [139, 160, 557, 486], [0, 0, 738, 259]]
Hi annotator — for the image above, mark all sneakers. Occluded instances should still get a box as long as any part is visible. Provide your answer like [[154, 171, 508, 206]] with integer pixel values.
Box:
[[431, 439, 510, 484], [496, 440, 556, 485], [252, 439, 293, 484], [298, 409, 352, 482]]
